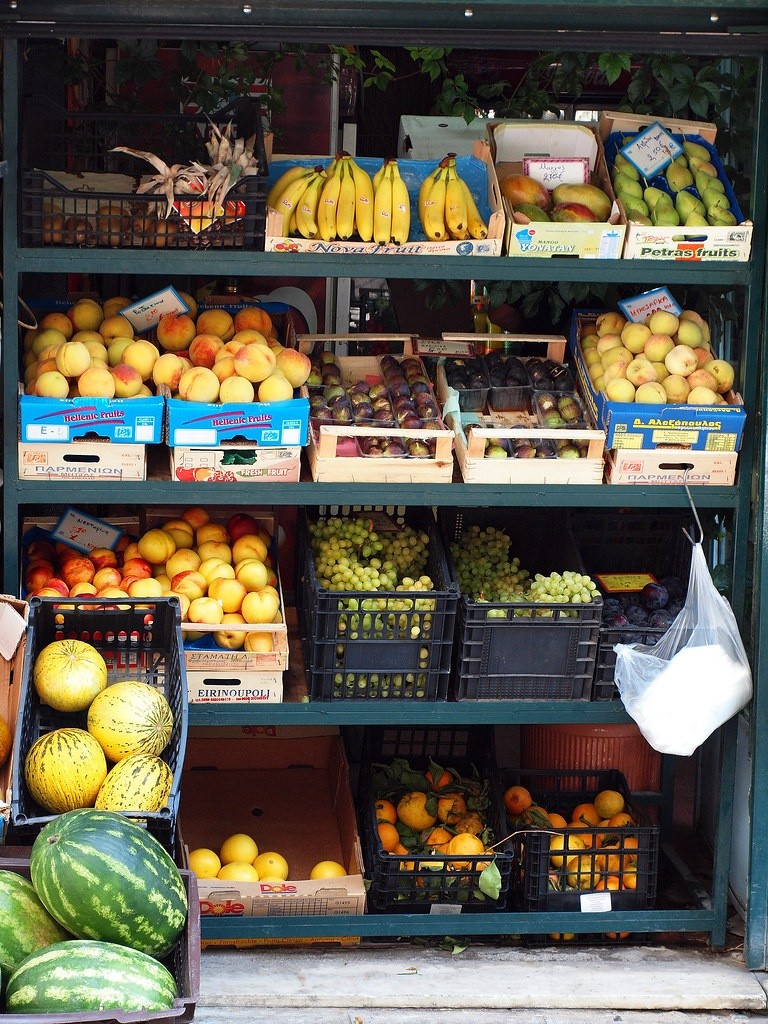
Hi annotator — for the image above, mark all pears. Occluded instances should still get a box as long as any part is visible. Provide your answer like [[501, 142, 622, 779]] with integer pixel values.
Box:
[[611, 127, 737, 226]]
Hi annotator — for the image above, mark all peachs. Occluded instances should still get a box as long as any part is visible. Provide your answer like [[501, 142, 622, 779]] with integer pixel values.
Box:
[[21, 506, 283, 653], [582, 310, 735, 405], [24, 292, 310, 403]]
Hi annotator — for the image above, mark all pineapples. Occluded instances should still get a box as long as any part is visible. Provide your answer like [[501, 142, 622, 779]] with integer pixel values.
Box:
[[448, 525, 602, 618]]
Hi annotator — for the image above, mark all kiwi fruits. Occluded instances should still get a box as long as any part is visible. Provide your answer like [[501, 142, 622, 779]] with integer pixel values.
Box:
[[43, 201, 176, 246]]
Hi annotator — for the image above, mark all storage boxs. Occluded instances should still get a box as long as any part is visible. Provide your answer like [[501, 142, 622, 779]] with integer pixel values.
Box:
[[486, 120, 627, 259], [18, 111, 269, 251], [599, 111, 753, 262], [264, 139, 506, 257], [0, 309, 747, 1024]]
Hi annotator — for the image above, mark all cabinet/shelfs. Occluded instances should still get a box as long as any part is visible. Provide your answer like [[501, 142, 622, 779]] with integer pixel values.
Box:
[[0, 22, 768, 948]]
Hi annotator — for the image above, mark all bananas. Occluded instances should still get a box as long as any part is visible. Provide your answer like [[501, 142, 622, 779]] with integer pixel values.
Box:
[[419, 152, 487, 241], [267, 151, 410, 246]]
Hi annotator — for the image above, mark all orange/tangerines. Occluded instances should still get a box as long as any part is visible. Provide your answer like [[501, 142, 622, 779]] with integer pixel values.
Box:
[[504, 786, 639, 939], [189, 835, 289, 882], [310, 861, 348, 880], [376, 770, 494, 888]]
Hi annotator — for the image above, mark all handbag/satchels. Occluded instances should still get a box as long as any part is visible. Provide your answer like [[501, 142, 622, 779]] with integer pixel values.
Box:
[[611, 544, 752, 755]]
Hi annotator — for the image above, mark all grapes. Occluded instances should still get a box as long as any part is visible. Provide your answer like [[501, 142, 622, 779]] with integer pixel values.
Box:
[[308, 519, 437, 698]]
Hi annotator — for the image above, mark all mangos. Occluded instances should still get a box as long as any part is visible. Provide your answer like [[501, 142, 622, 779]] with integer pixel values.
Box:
[[499, 173, 611, 223]]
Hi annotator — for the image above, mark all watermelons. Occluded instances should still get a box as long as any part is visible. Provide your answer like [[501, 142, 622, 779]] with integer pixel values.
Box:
[[31, 808, 187, 959], [6, 940, 179, 1014], [0, 869, 72, 981]]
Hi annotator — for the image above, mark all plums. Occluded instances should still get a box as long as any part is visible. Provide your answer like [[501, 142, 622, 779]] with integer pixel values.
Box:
[[602, 576, 687, 645]]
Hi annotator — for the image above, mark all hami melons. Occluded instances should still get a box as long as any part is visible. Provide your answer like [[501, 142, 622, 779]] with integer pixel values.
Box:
[[25, 638, 173, 822]]
